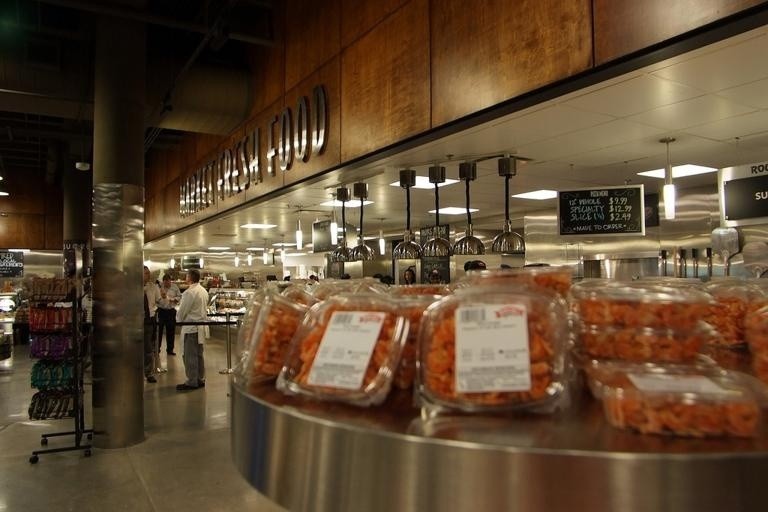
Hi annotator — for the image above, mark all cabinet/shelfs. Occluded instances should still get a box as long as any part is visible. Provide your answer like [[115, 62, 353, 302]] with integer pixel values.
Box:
[[20, 280, 97, 466]]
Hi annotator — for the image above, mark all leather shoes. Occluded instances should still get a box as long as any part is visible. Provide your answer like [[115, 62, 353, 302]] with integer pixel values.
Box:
[[146, 368, 167, 382], [176, 379, 204, 390], [167, 351, 176, 355]]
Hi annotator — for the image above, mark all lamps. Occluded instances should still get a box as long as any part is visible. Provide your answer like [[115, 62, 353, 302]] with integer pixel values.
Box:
[[658, 136, 680, 221], [328, 156, 526, 263], [293, 203, 305, 251]]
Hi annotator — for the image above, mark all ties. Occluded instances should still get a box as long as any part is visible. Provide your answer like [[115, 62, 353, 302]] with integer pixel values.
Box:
[[144, 292, 151, 320]]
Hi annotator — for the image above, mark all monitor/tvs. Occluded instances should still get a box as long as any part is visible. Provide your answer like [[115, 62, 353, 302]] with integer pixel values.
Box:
[[310, 219, 338, 253], [645, 192, 660, 228]]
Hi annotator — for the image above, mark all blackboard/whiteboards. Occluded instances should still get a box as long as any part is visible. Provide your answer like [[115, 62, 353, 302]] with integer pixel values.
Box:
[[557, 183, 646, 238]]
